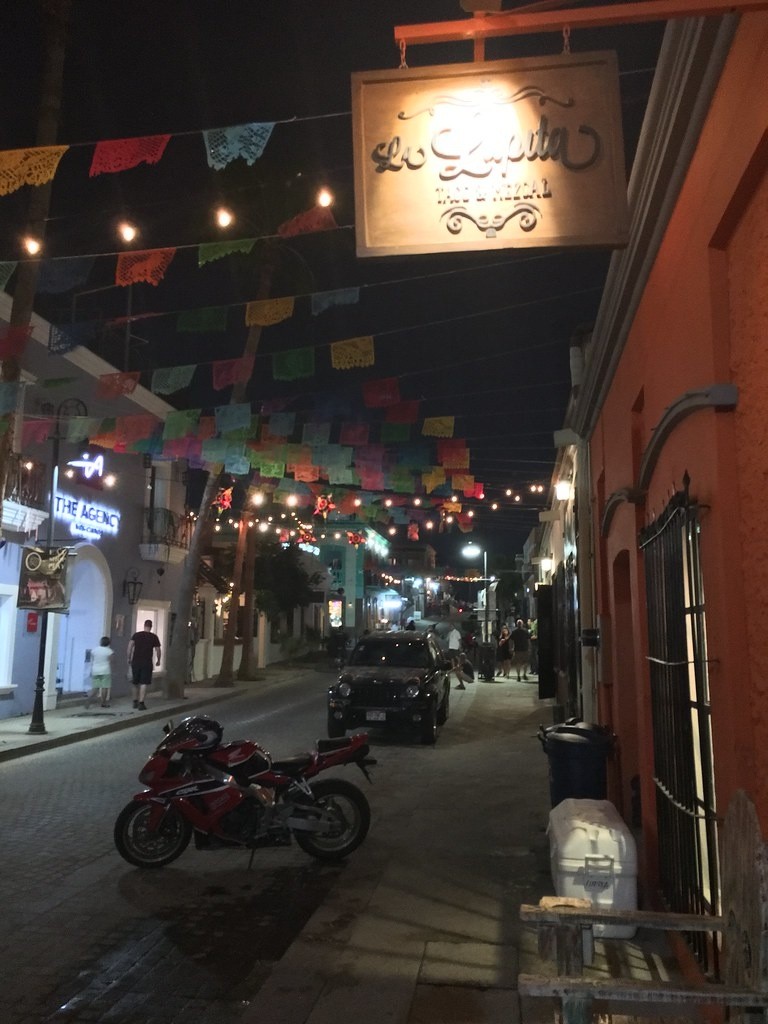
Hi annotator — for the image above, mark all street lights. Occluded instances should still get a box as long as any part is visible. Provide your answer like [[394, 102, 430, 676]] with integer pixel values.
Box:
[[461, 540, 494, 681], [413, 575, 431, 617], [25, 397, 90, 737]]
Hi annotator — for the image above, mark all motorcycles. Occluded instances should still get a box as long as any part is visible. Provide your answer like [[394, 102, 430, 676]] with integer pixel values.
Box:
[[114, 712, 378, 869]]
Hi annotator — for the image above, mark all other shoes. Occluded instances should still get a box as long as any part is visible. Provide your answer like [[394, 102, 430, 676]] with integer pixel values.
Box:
[[523, 674, 528, 680], [456, 685, 465, 690], [529, 671, 532, 674], [132, 700, 138, 708], [533, 671, 537, 675], [516, 676, 521, 682], [137, 702, 147, 710], [102, 704, 110, 707]]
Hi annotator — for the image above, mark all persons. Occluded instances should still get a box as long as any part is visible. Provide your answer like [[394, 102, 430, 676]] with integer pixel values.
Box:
[[451, 653, 475, 690], [496, 617, 536, 682], [405, 620, 416, 630], [446, 623, 462, 671], [427, 628, 435, 638], [127, 620, 162, 710], [85, 636, 114, 709]]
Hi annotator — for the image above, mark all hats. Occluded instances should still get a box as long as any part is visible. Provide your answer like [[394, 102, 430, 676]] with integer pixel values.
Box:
[[516, 619, 524, 628]]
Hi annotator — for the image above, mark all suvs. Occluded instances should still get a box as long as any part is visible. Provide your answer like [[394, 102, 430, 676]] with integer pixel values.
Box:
[[327, 630, 452, 744]]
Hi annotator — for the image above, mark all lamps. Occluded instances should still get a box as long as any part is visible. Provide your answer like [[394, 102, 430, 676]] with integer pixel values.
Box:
[[122, 567, 144, 605]]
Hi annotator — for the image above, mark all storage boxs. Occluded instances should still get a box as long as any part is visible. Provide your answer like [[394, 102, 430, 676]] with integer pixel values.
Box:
[[545, 799, 638, 939]]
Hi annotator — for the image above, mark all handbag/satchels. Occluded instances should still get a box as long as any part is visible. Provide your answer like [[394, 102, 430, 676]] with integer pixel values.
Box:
[[126, 665, 134, 681]]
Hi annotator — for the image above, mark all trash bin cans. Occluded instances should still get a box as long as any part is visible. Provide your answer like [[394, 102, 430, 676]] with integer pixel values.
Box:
[[536, 718, 610, 807]]
